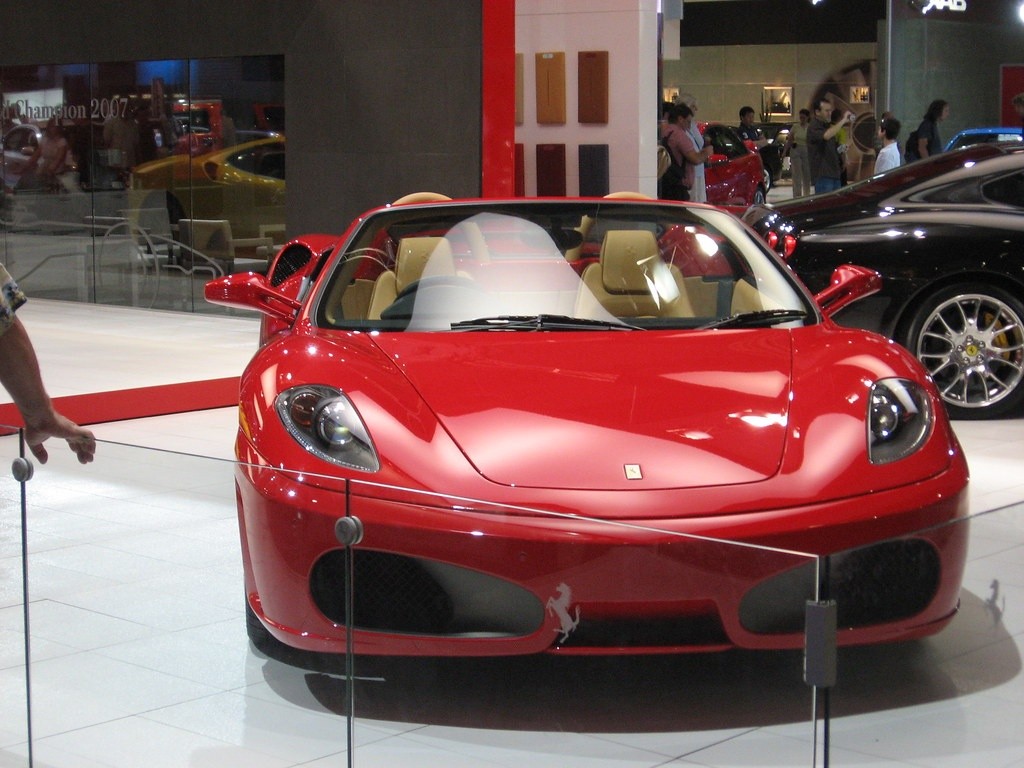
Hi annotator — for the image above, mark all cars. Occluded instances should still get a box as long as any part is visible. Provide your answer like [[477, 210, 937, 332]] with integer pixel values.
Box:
[[738, 124, 1024, 424]]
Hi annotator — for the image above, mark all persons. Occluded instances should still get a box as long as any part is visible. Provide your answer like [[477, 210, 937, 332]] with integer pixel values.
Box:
[[829, 110, 849, 188], [0, 262, 97, 464], [19, 93, 185, 190], [659, 92, 715, 202], [916, 99, 949, 159], [738, 107, 758, 141], [873, 112, 900, 176], [806, 98, 852, 195], [781, 109, 811, 198]]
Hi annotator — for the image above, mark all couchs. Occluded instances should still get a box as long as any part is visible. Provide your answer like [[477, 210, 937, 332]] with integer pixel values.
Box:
[[115, 208, 286, 277]]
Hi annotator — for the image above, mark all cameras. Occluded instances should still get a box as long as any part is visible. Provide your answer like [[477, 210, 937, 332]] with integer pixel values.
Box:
[[849, 115, 856, 121]]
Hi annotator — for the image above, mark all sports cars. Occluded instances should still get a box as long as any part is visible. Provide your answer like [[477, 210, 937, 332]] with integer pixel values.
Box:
[[199, 189, 973, 670], [695, 120, 771, 209], [0, 99, 284, 263]]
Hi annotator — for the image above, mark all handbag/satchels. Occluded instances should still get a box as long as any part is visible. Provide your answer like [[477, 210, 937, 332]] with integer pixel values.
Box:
[[661, 130, 687, 184]]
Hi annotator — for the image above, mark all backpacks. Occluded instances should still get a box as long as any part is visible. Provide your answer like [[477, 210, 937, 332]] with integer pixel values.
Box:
[[904, 123, 932, 161]]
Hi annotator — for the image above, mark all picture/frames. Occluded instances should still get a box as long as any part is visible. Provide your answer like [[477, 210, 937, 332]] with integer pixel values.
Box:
[[763, 86, 792, 116]]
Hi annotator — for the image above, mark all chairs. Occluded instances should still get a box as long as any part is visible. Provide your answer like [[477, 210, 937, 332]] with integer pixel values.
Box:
[[368, 236, 476, 320], [575, 230, 696, 317]]
